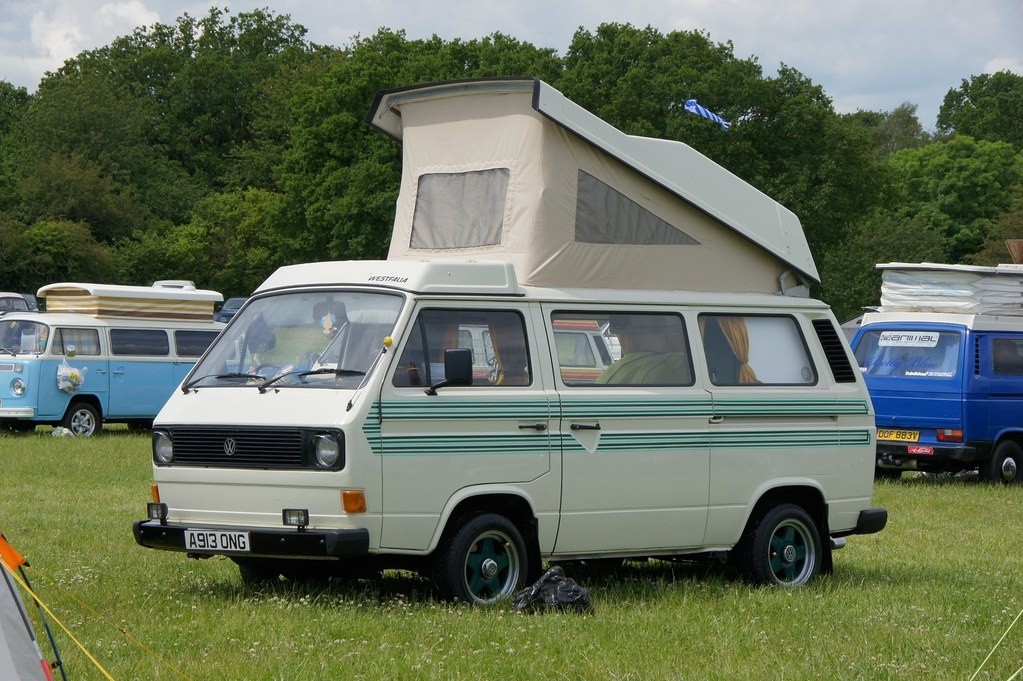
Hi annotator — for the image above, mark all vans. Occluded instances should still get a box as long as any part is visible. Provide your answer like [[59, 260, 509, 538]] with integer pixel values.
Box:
[[0, 282, 228, 441], [131, 76, 889, 613], [0, 292, 30, 331], [851, 321, 1023, 485]]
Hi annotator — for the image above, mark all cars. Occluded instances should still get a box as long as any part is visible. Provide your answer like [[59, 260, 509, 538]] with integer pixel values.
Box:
[[21, 293, 41, 312], [217, 297, 254, 326]]
